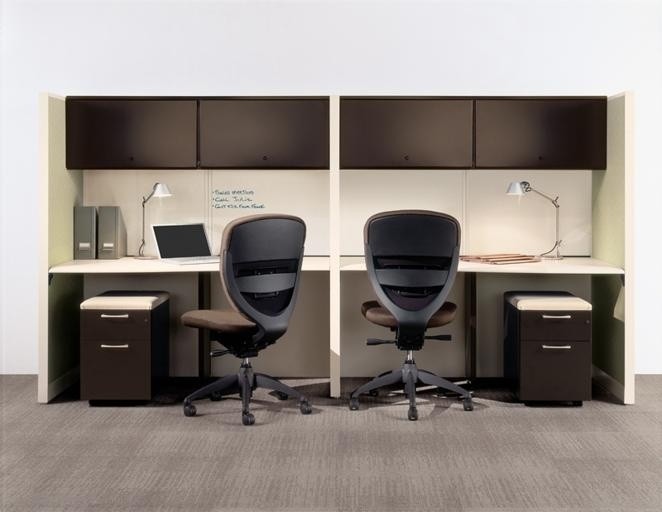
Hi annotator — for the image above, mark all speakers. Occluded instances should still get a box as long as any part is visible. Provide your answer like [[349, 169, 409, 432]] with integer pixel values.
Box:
[[74, 206, 96, 259], [98, 205, 127, 258]]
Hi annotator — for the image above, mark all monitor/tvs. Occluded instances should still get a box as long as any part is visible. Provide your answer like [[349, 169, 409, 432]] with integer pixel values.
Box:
[[151, 222, 221, 265]]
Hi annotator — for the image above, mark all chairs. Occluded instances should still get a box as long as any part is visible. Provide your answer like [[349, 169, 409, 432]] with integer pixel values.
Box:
[[347, 209, 473, 420], [180, 216, 312, 426]]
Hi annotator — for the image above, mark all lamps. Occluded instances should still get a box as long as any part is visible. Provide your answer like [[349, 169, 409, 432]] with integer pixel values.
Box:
[[506, 181, 562, 260]]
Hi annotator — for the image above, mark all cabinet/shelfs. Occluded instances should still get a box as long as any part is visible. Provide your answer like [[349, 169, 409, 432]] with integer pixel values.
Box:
[[340, 98, 475, 168], [38, 92, 635, 405], [66, 96, 198, 169], [475, 98, 608, 168], [199, 98, 329, 168], [81, 289, 170, 406], [504, 290, 593, 408]]
